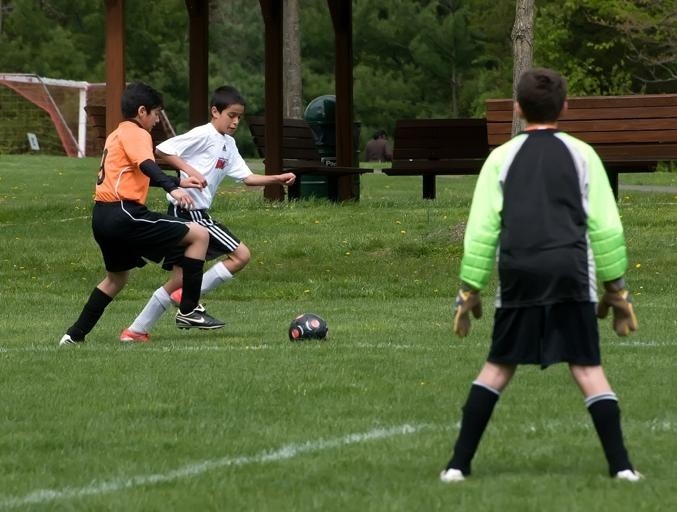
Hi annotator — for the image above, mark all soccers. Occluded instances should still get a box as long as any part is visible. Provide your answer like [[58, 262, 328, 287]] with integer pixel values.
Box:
[[288, 313, 328, 342]]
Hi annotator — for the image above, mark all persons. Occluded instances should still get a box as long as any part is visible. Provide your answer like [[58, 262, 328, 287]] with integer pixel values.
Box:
[[440, 69, 646, 481], [363, 130, 394, 161], [60, 83, 225, 346], [120, 85, 296, 341]]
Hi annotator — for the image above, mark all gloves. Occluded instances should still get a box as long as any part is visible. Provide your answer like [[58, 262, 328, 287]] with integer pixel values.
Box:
[[453, 284, 484, 338], [599, 291, 638, 340]]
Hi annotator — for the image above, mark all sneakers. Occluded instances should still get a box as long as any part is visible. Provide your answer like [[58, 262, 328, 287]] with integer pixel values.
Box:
[[169, 286, 185, 308], [437, 466, 474, 486], [613, 468, 647, 485], [118, 327, 156, 344], [59, 334, 82, 351], [173, 304, 228, 332]]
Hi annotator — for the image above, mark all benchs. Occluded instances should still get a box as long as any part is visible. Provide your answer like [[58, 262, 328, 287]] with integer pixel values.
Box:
[[382, 118, 487, 199], [485, 92, 677, 204], [245, 116, 374, 205]]
